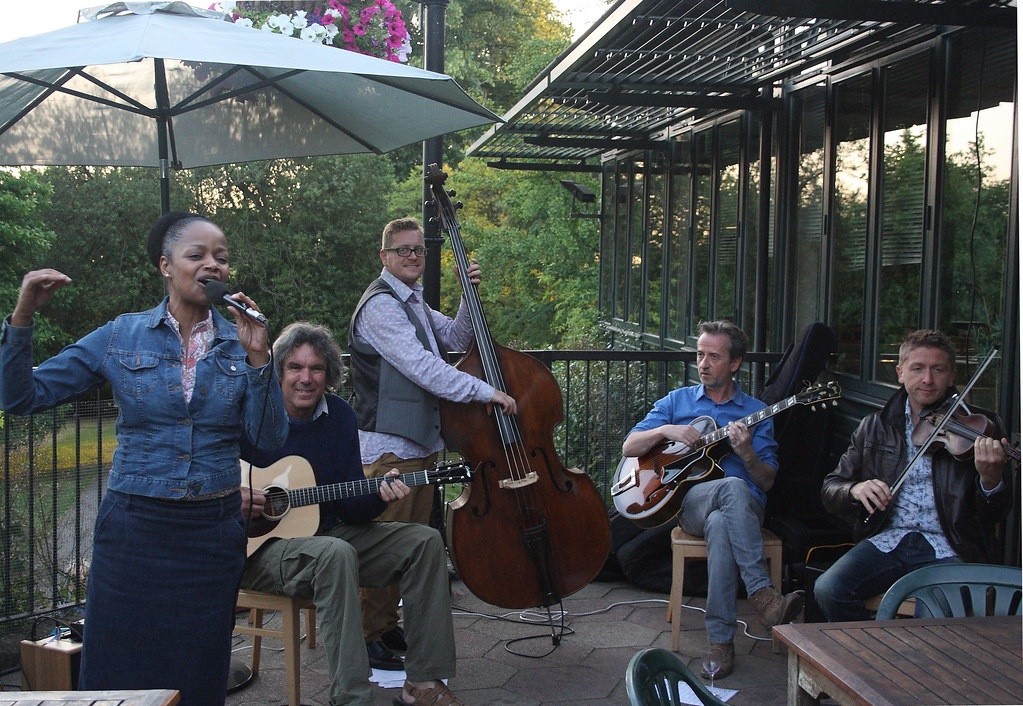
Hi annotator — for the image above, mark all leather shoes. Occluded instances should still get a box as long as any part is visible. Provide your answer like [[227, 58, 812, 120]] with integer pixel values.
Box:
[[366, 641, 404, 671], [381, 629, 407, 655]]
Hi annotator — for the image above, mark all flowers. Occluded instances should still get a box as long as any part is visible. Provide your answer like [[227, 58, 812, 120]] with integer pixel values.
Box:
[[179, 0, 414, 107]]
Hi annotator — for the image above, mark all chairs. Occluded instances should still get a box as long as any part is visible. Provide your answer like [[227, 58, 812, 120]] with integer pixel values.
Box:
[[662, 521, 785, 656], [798, 539, 918, 619], [236, 586, 321, 706], [870, 561, 1022, 619], [623, 646, 733, 706]]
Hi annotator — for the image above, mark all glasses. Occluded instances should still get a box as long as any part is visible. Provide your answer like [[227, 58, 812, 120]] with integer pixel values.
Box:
[[383, 247, 430, 258]]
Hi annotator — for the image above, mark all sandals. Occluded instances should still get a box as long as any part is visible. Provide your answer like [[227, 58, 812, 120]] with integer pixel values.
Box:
[[393, 679, 465, 706]]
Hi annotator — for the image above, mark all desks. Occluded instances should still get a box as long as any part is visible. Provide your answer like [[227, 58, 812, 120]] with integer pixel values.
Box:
[[764, 612, 1023, 706]]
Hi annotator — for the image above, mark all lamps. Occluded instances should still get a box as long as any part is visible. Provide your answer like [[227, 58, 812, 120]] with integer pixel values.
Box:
[[559, 179, 600, 218]]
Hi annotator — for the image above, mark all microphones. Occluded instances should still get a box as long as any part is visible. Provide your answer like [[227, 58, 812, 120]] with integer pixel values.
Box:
[[203, 281, 268, 325]]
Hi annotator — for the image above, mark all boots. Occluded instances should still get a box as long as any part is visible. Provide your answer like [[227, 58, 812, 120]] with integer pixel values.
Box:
[[751, 587, 803, 635], [701, 642, 735, 679]]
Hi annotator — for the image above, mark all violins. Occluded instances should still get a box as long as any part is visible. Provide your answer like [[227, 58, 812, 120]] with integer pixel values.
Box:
[[910, 397, 1021, 471]]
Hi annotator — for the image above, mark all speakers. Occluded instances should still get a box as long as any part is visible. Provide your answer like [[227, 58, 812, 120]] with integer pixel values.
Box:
[[19, 616, 86, 693]]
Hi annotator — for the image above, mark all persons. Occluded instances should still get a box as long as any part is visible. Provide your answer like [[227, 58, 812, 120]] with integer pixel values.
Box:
[[0, 209, 288, 706], [241, 321, 466, 706], [815, 329, 1009, 623], [621, 320, 805, 679], [350, 219, 518, 670]]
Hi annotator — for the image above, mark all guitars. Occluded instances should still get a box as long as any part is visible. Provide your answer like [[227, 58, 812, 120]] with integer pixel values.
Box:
[[240, 455, 484, 558], [610, 378, 842, 532]]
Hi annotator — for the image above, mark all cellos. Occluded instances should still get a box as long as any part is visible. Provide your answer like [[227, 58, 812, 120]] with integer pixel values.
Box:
[[423, 159, 614, 647]]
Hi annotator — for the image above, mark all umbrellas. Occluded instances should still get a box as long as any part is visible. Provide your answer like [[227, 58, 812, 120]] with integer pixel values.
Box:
[[0, 0, 508, 217]]
